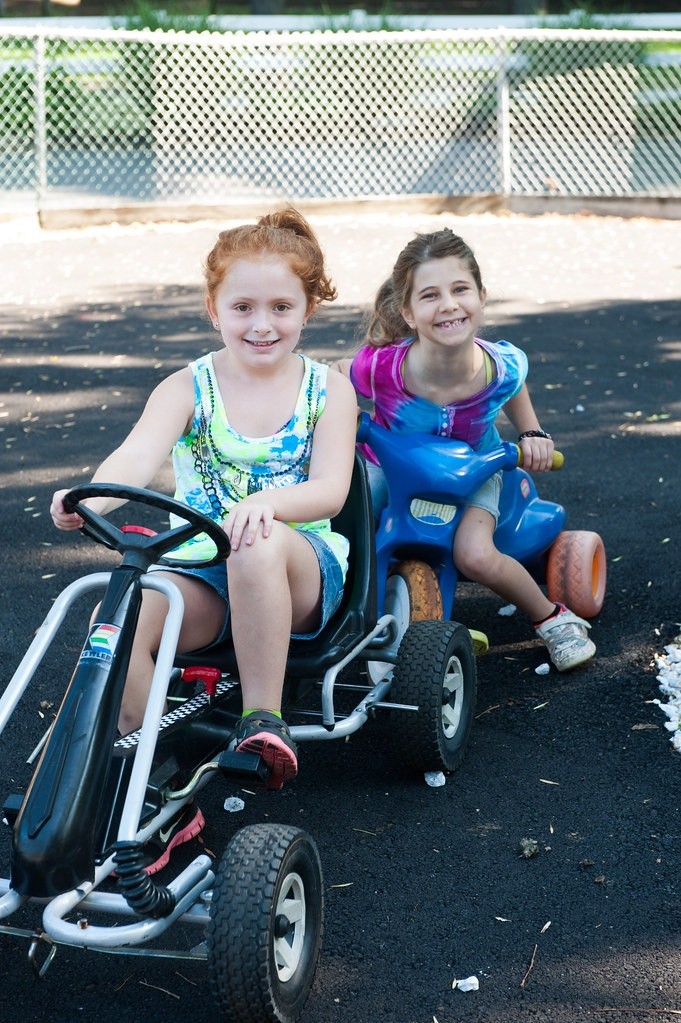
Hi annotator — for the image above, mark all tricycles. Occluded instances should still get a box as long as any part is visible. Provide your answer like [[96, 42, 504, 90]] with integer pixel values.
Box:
[[348, 411, 608, 624]]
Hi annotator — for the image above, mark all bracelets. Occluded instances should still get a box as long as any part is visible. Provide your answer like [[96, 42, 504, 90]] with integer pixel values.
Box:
[[519, 430, 552, 441]]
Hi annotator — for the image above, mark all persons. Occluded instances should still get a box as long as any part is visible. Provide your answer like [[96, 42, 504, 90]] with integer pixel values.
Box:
[[48, 206, 359, 878], [331, 230, 598, 670]]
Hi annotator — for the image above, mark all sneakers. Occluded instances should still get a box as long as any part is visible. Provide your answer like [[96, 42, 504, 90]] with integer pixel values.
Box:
[[532, 602, 596, 672], [109, 805, 206, 877], [232, 710, 298, 792]]
